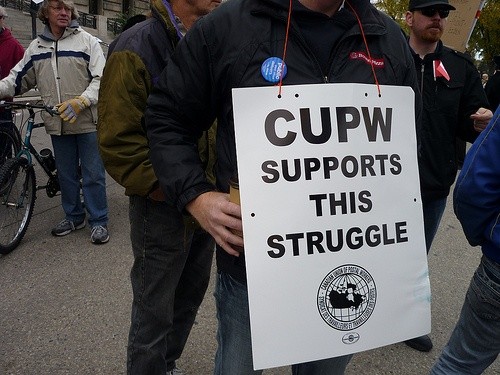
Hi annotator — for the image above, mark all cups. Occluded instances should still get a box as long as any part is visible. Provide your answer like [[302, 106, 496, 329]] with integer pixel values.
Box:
[[228, 176, 244, 250], [39, 147, 56, 174]]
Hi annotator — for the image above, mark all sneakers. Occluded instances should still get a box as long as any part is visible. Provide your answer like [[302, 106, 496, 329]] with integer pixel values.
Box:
[[51, 218, 86, 236], [90, 225, 109, 244]]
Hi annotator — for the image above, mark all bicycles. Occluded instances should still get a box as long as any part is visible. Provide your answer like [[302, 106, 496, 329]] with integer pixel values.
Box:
[[0, 101, 22, 197], [0, 99, 100, 255]]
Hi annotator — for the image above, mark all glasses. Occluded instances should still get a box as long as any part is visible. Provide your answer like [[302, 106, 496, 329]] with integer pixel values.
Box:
[[415, 8, 449, 19]]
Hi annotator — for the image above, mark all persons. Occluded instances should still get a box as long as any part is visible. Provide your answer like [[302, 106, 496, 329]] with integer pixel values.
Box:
[[146, 0, 424, 375], [94, 0, 224, 375], [1, 0, 111, 246], [429, 97, 500, 375], [107, 14, 147, 61], [476, 61, 500, 111], [397, 0, 493, 258], [0, 5, 28, 196]]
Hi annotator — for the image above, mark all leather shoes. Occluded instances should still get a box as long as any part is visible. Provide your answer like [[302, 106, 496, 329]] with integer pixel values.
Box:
[[403, 335, 433, 353]]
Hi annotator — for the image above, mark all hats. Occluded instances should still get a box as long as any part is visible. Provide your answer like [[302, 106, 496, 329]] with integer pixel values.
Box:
[[409, 0, 456, 12]]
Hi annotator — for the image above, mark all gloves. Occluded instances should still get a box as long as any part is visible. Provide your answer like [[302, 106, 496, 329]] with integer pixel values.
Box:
[[55, 96, 88, 124]]
